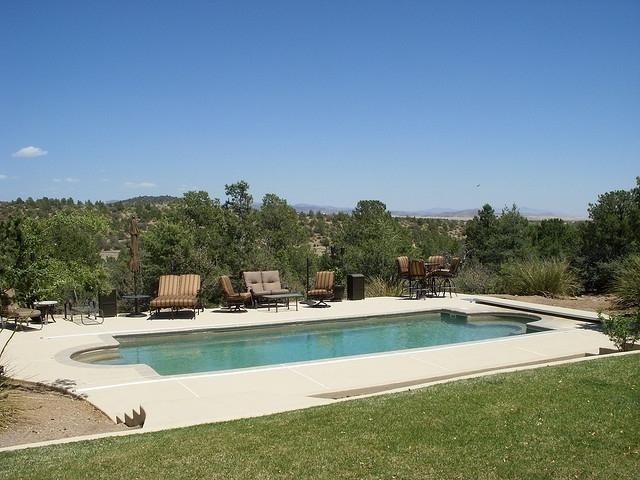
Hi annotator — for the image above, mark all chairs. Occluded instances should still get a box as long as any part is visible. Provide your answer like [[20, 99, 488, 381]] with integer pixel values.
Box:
[[3, 285, 104, 331], [395, 255, 461, 300], [217, 275, 252, 312], [307, 271, 335, 308]]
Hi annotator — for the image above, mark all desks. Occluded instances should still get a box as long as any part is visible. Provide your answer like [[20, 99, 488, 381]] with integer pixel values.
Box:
[[262, 293, 305, 312], [120, 294, 152, 318]]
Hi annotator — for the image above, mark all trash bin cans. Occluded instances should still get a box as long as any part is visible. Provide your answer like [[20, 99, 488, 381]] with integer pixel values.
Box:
[[98, 288, 117, 317], [346, 274, 364, 300]]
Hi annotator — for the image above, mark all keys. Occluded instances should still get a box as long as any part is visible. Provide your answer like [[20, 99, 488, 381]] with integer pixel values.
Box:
[[128, 215, 141, 313]]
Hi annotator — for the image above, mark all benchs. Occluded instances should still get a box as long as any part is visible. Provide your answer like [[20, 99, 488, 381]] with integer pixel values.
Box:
[[241, 270, 292, 309], [149, 274, 205, 319]]
